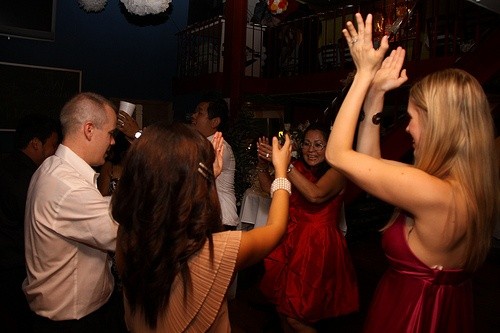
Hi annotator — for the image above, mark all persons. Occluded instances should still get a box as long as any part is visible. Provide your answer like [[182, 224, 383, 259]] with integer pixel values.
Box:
[[117, 92, 269, 333], [21, 92, 131, 333], [0, 113, 58, 333], [267, 0, 322, 76], [109, 122, 292, 333], [256, 123, 360, 333], [324, 13, 500, 333]]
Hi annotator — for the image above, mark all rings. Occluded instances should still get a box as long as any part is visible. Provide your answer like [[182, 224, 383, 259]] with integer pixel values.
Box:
[[266, 153, 269, 158]]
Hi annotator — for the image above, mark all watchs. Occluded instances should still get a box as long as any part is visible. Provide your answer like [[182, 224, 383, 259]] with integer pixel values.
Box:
[[134, 129, 143, 140]]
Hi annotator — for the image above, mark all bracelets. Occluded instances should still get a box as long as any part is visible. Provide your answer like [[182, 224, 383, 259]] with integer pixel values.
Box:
[[270, 178, 292, 198], [287, 164, 294, 174], [360, 108, 382, 126]]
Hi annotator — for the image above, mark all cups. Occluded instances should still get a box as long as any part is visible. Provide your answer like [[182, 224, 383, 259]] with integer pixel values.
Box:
[[118, 101, 135, 123]]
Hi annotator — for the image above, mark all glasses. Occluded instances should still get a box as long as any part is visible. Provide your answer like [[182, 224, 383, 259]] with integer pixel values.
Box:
[[300, 141, 325, 151]]
